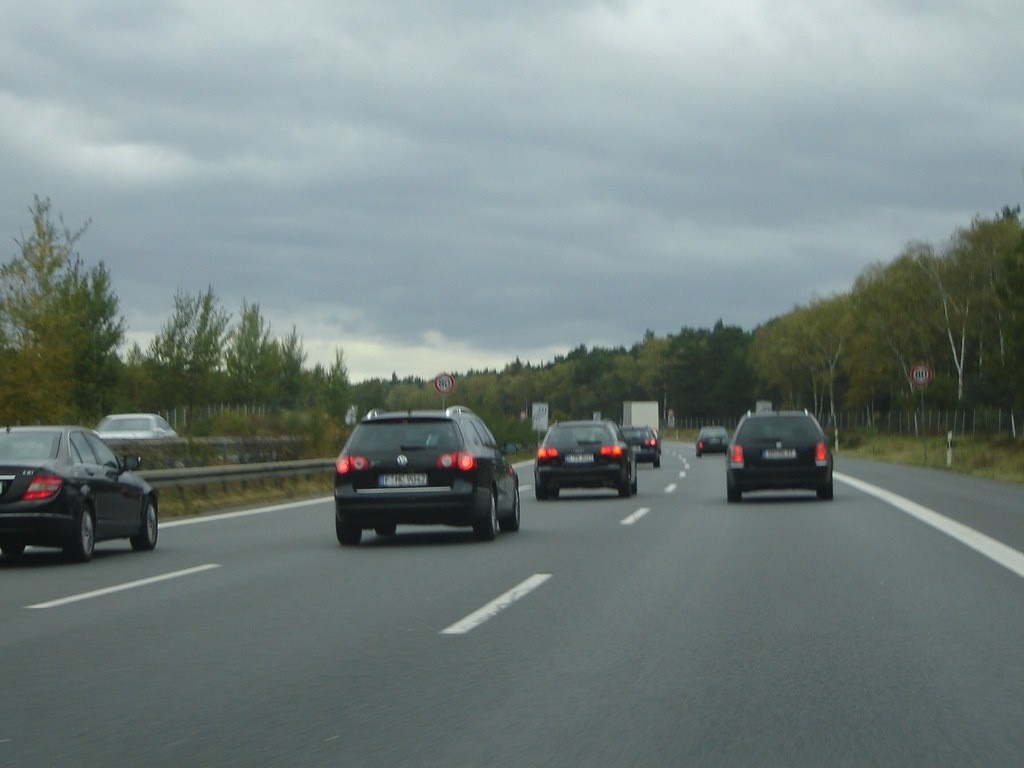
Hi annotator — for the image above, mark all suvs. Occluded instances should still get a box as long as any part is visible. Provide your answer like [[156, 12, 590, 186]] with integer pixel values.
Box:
[[534, 421, 637, 500], [727, 409, 834, 503], [334, 405, 520, 545]]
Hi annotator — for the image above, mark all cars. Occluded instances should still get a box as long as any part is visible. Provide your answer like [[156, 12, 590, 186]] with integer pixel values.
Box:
[[619, 424, 661, 469], [696, 425, 727, 457], [94, 412, 177, 440], [0, 424, 158, 562]]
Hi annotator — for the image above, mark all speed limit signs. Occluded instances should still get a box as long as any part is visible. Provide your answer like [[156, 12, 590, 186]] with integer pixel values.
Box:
[[911, 364, 932, 386], [434, 373, 456, 393]]
